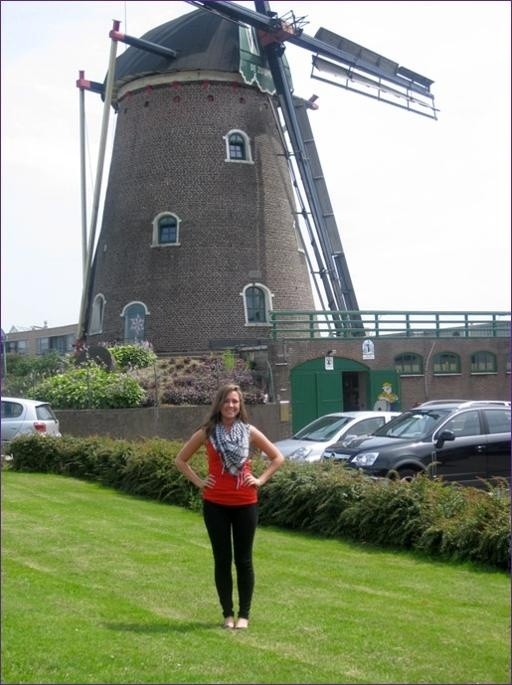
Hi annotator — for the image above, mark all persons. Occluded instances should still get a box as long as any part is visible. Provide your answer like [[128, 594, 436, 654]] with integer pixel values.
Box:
[[176, 384, 284, 629]]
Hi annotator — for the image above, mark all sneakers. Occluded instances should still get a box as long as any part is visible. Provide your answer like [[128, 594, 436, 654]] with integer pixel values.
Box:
[[224, 616, 234, 628], [237, 619, 249, 629]]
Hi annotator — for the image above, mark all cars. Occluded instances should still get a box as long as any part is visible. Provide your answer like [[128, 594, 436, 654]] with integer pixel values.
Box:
[[0, 396, 62, 449], [261, 399, 512, 495]]
